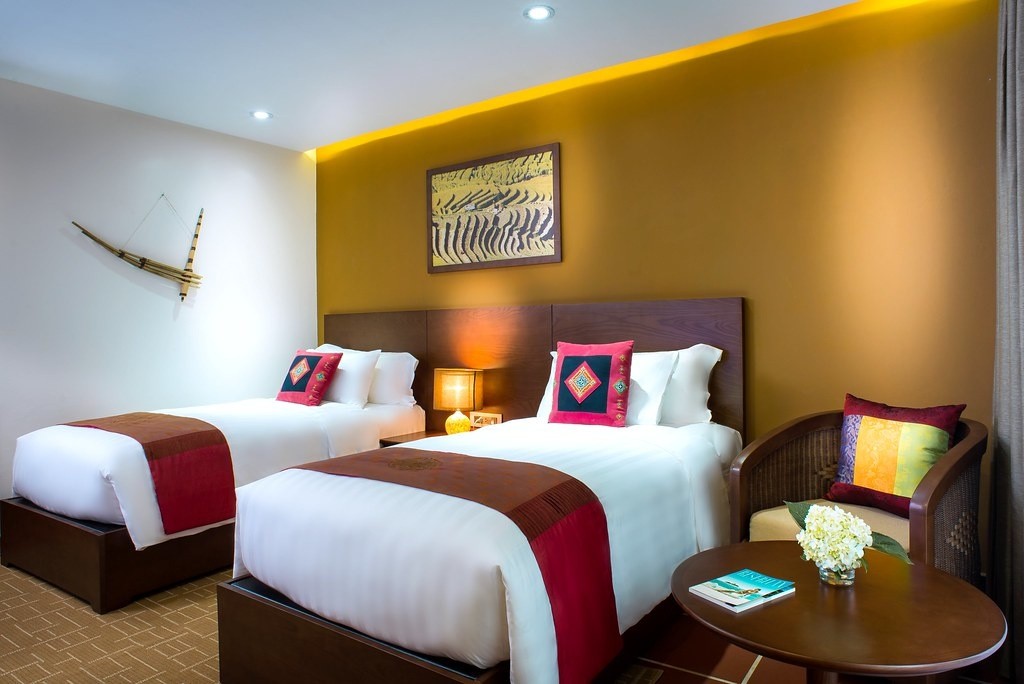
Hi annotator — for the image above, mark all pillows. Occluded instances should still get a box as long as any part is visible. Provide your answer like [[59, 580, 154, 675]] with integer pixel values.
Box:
[[369, 353, 418, 408], [824, 393, 967, 517], [548, 341, 634, 426], [537, 352, 679, 425], [306, 348, 381, 410], [661, 343, 724, 426], [277, 350, 343, 406]]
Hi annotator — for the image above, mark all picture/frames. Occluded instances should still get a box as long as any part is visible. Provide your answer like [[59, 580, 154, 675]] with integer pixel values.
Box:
[[426, 142, 562, 273]]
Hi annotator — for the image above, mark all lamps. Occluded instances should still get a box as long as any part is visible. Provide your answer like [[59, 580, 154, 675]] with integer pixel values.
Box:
[[433, 365, 485, 434]]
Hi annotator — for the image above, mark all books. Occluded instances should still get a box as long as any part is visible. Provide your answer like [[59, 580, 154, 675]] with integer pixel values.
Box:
[[689, 569, 795, 614]]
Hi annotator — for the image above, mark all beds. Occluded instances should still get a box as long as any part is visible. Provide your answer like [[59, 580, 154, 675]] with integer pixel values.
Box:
[[218, 298, 744, 684], [0, 312, 426, 612]]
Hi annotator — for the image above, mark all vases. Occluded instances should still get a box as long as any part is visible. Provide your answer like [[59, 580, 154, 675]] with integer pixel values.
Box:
[[820, 569, 856, 585]]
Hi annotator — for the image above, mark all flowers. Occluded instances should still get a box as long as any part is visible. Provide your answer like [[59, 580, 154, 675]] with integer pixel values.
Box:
[[782, 501, 914, 572]]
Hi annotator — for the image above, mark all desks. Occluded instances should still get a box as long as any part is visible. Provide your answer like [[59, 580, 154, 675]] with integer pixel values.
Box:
[[377, 426, 483, 449], [671, 539, 1008, 684]]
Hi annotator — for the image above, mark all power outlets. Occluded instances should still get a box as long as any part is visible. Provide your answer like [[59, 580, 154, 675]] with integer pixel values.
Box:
[[470, 412, 502, 427]]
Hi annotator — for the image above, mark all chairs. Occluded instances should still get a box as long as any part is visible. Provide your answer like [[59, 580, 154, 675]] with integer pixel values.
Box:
[[727, 407, 988, 684]]
